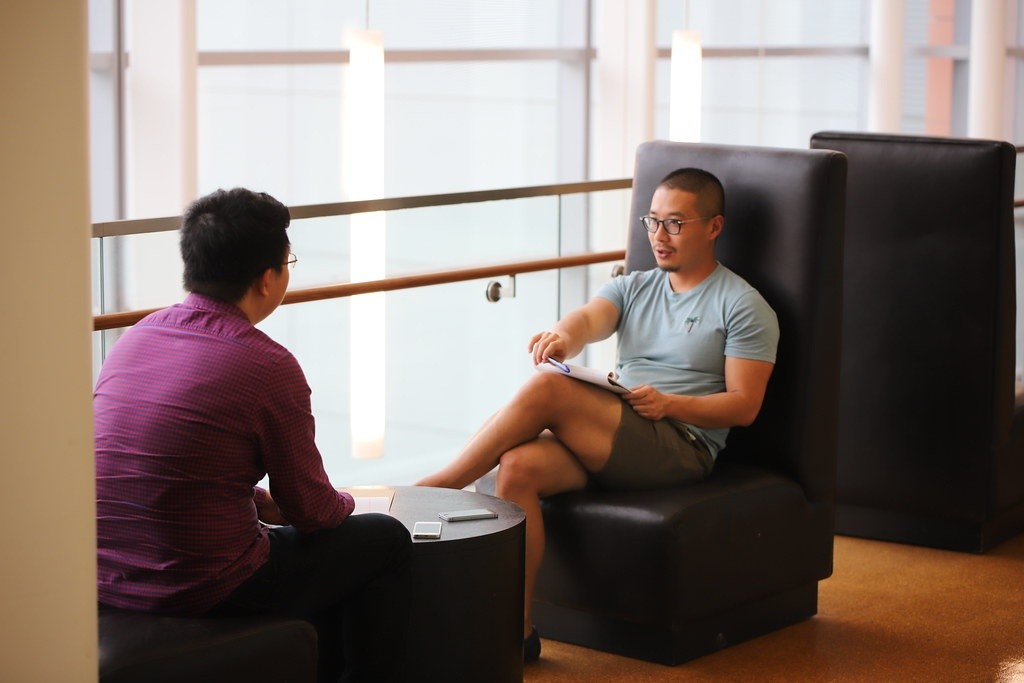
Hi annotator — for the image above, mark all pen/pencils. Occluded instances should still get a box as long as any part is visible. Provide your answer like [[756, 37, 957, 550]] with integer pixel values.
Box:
[[547, 356, 570, 373]]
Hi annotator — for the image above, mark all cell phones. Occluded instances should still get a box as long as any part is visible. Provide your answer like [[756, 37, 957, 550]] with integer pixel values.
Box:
[[413, 522, 442, 539], [437, 508, 498, 521]]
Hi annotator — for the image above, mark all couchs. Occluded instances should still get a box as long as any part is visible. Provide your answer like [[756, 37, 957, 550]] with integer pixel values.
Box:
[[476, 142, 849, 666], [811, 132, 1024, 553], [97, 601, 318, 683]]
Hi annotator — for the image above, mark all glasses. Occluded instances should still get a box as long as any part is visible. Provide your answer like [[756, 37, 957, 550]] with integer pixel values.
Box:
[[271, 252, 297, 272], [639, 215, 712, 235]]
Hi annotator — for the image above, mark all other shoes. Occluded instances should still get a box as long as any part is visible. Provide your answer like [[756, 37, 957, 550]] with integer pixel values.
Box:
[[523, 625, 541, 664]]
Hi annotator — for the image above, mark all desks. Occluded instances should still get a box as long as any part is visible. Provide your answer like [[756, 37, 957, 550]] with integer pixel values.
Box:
[[347, 486, 527, 683]]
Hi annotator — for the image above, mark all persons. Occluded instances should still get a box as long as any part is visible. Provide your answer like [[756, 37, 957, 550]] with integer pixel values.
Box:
[[91, 188, 416, 683], [413, 169, 779, 662]]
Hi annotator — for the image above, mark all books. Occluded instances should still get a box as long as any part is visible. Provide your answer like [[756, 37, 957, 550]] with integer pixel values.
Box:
[[535, 363, 696, 444]]
